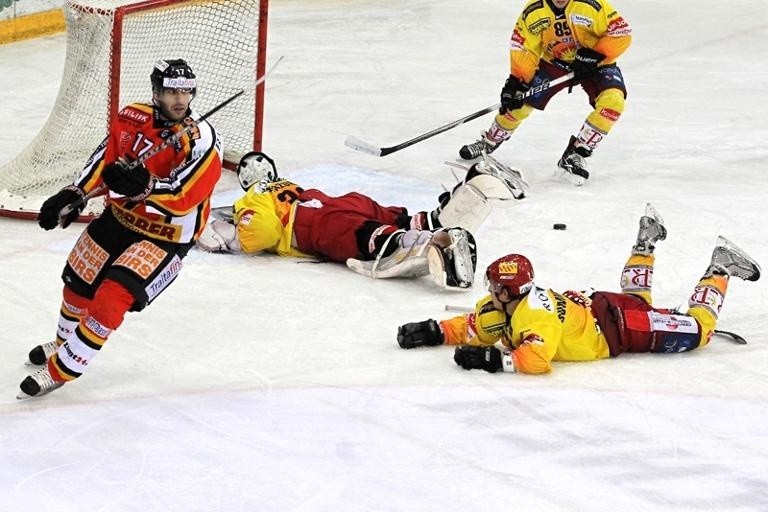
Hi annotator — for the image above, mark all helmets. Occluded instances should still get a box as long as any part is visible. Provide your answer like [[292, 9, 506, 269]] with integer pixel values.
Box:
[[150, 59, 197, 98], [482, 255, 535, 298], [237, 152, 277, 191]]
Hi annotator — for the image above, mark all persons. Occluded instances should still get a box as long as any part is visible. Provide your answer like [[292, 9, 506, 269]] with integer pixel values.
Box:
[[397, 216, 761, 375], [19, 57, 223, 397], [459, 1, 633, 179], [228, 152, 531, 291]]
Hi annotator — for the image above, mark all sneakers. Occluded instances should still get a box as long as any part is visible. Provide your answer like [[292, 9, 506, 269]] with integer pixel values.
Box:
[[28, 342, 62, 365], [460, 130, 589, 200], [447, 227, 474, 283], [20, 365, 64, 397]]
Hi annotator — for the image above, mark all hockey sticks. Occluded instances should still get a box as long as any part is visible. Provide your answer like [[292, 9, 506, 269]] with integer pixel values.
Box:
[[445, 304, 746, 346], [344, 72, 574, 157], [57, 55, 285, 216]]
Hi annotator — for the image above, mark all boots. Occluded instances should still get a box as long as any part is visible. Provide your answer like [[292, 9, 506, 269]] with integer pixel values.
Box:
[[631, 216, 666, 256], [700, 247, 760, 290]]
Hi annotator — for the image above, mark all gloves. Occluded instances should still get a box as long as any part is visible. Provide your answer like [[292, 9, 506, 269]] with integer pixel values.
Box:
[[37, 185, 88, 231], [570, 46, 606, 88], [102, 154, 156, 203], [396, 319, 445, 348], [454, 344, 502, 373], [500, 73, 529, 116]]
